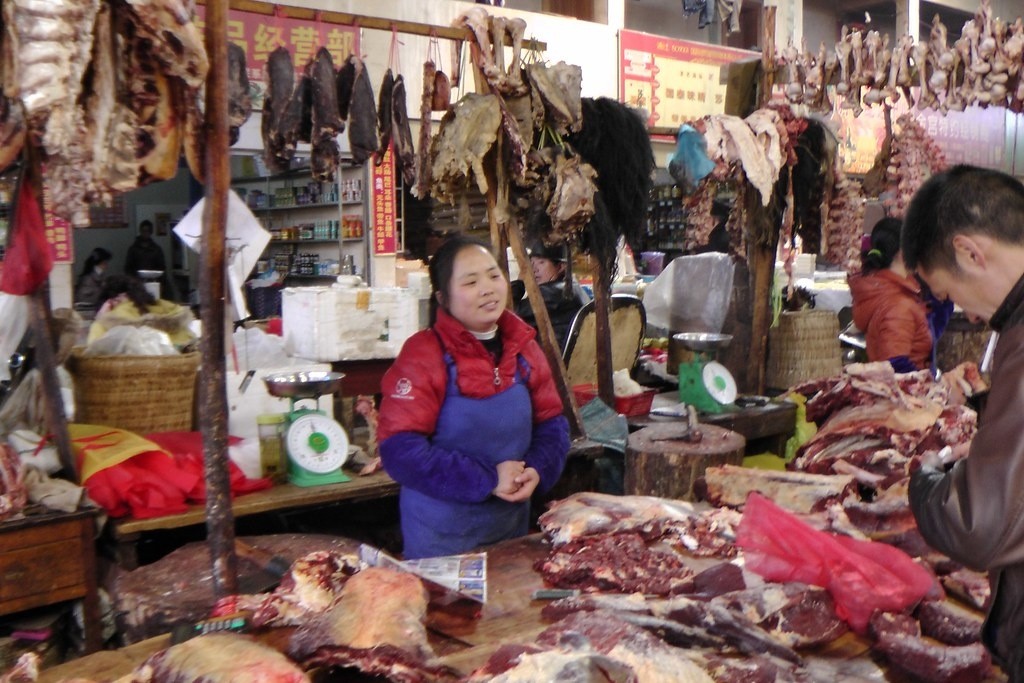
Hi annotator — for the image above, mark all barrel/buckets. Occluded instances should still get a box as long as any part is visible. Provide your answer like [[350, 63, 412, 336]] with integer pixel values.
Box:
[[636, 275, 655, 283], [641, 252, 666, 275]]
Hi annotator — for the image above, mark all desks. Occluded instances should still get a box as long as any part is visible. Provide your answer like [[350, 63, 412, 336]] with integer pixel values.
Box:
[[0, 505, 105, 656], [111, 470, 393, 569], [331, 359, 393, 422], [622, 387, 808, 457], [35, 535, 554, 683]]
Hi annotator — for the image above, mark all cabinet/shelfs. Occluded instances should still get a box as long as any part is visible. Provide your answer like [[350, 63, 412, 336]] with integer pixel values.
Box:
[[232, 164, 362, 279]]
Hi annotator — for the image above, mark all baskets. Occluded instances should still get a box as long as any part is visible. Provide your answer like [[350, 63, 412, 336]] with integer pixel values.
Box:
[[67, 346, 203, 433]]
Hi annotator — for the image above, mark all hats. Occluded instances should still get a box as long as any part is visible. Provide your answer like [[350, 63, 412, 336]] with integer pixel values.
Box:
[[527, 238, 576, 264]]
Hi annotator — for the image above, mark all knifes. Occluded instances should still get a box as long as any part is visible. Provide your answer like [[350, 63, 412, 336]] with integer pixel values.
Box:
[[532, 590, 660, 598]]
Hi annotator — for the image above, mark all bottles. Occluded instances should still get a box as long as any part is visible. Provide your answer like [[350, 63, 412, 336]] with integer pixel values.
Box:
[[644, 181, 685, 241], [343, 254, 352, 275]]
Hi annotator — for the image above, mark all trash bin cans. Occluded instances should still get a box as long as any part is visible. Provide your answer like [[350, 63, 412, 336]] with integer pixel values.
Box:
[[641, 252, 665, 276], [246, 287, 282, 319]]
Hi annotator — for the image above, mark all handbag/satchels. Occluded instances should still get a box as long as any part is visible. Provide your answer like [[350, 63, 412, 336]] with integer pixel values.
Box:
[[735, 491, 931, 635], [66, 424, 275, 518], [668, 124, 717, 194], [0, 188, 56, 296]]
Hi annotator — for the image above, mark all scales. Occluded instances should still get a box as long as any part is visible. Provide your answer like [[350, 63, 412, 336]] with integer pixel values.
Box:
[[673, 332, 737, 413], [264, 371, 351, 488], [137, 270, 164, 299]]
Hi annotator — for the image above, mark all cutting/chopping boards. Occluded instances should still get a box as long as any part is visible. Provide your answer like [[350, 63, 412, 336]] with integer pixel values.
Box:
[[624, 421, 746, 504], [110, 533, 362, 643], [939, 331, 994, 385]]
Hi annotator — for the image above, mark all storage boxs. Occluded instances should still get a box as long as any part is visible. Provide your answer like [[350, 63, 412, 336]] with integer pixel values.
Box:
[[230, 254, 433, 470]]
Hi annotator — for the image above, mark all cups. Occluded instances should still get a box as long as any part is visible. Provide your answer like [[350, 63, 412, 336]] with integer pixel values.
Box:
[[258, 413, 288, 483]]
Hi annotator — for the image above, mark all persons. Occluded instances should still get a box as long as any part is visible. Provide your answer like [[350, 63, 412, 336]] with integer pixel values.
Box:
[[86, 274, 197, 345], [900, 163, 1024, 683], [74, 247, 112, 311], [510, 233, 584, 350], [847, 218, 933, 374], [377, 236, 571, 560], [125, 220, 173, 300]]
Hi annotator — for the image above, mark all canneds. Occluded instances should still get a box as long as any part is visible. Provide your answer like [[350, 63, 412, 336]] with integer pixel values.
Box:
[[273, 253, 319, 275], [271, 179, 361, 207], [270, 219, 338, 240]]
